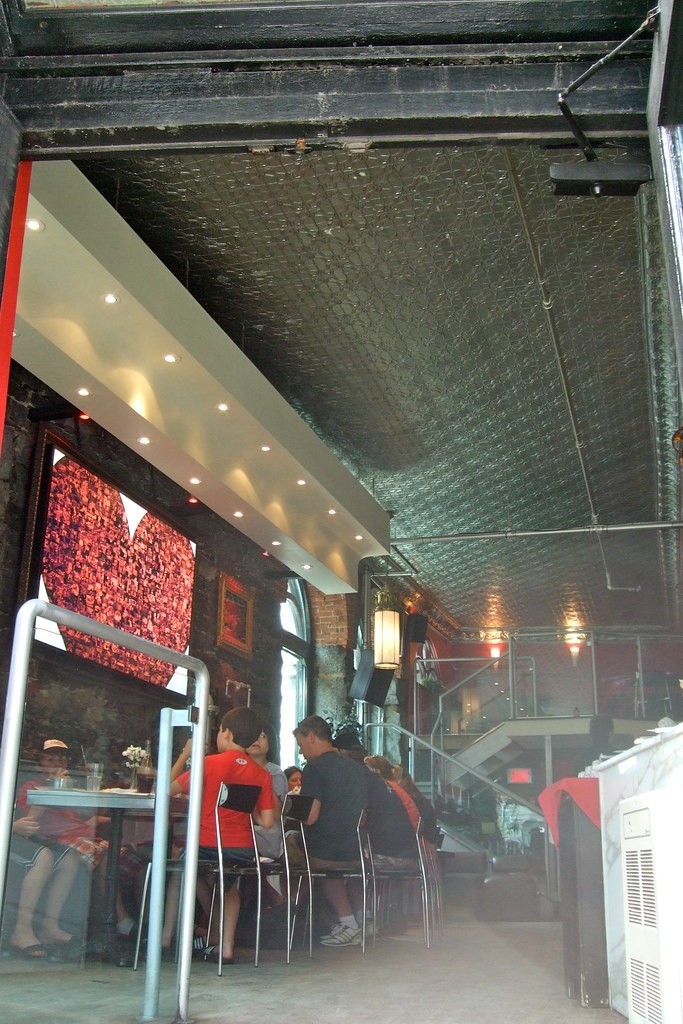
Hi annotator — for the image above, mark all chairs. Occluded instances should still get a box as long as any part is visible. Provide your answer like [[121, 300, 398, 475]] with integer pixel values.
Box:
[[130, 782, 263, 976], [634, 669, 672, 720], [289, 807, 378, 954], [204, 795, 315, 964], [372, 814, 446, 949]]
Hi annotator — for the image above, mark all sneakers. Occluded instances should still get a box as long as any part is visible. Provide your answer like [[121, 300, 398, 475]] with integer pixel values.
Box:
[[361, 918, 378, 939], [318, 920, 363, 948]]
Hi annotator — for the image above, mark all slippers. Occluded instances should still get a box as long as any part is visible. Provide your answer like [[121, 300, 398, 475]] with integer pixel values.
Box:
[[5, 938, 50, 962], [141, 935, 169, 955], [199, 943, 233, 964], [49, 933, 84, 952]]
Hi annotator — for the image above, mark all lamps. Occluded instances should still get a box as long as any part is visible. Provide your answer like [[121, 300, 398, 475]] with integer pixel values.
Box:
[[372, 556, 404, 671]]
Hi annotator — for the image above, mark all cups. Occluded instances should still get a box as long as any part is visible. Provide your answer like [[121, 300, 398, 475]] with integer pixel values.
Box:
[[137, 767, 155, 792], [86, 763, 104, 792]]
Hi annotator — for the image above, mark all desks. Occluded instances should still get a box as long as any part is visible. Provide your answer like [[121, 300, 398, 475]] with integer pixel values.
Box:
[[25, 790, 189, 968]]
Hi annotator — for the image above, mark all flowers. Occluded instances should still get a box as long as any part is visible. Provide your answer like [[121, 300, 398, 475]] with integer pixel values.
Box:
[[121, 744, 152, 789], [183, 747, 191, 771]]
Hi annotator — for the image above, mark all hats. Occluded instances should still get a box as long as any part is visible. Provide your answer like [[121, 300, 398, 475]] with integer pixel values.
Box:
[[39, 738, 72, 753]]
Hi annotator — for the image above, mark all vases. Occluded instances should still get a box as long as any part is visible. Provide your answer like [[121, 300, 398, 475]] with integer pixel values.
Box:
[[127, 765, 139, 791]]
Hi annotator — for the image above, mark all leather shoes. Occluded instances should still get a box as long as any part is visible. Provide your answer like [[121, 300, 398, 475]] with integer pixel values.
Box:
[[114, 922, 146, 966]]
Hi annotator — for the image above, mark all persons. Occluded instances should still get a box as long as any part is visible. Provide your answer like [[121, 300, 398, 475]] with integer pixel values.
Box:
[[6, 704, 443, 965], [574, 715, 621, 777]]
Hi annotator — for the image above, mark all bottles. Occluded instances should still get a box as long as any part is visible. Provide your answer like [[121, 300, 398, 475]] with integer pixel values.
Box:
[[136, 739, 155, 766]]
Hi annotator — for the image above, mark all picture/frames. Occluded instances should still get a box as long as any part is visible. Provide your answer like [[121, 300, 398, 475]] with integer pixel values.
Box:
[[218, 571, 255, 661]]
